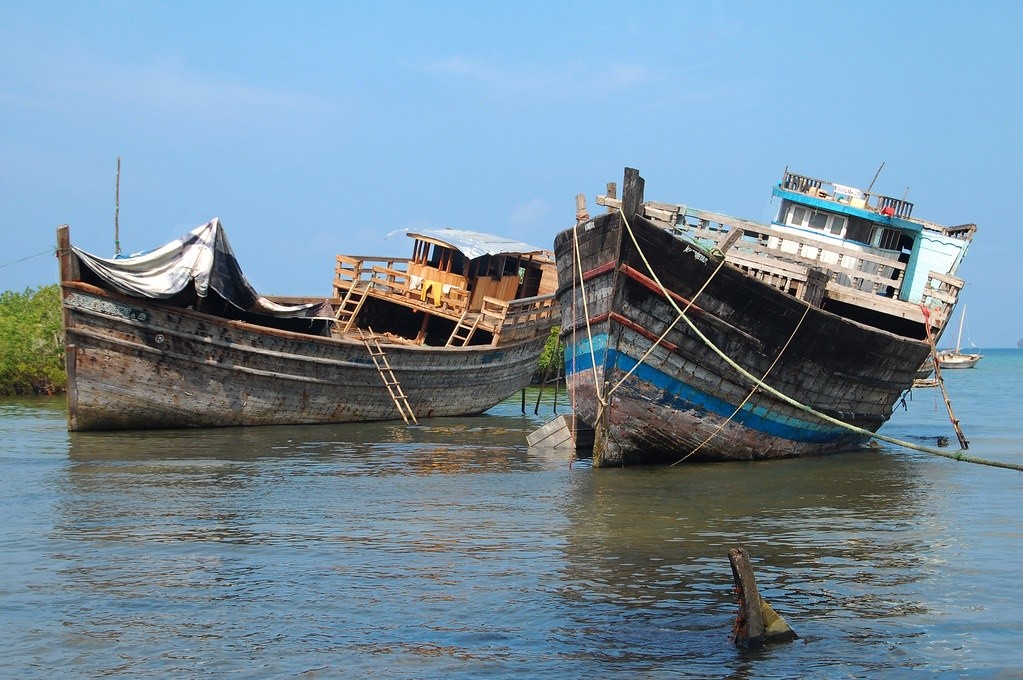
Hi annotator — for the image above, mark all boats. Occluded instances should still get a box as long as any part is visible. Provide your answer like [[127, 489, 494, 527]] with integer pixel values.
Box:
[[55, 223, 561, 433], [916, 352, 935, 378], [915, 378, 940, 388], [553, 165, 976, 467]]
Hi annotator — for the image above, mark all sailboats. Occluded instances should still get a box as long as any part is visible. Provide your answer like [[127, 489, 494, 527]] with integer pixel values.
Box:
[[937, 303, 985, 371]]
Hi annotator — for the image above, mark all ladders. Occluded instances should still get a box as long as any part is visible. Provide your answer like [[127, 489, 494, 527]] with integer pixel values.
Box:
[[331, 276, 372, 333], [445, 310, 483, 347], [357, 325, 419, 426]]
